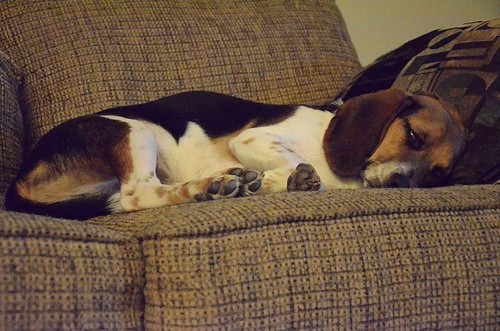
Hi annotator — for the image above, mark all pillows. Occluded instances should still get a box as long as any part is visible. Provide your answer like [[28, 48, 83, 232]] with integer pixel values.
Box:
[[332, 16, 500, 186]]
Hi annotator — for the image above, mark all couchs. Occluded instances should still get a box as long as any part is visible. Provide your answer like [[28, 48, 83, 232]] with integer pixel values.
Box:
[[0, 0, 500, 331]]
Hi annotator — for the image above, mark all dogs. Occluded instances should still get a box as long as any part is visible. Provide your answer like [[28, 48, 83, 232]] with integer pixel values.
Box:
[[4, 90, 467, 220]]
